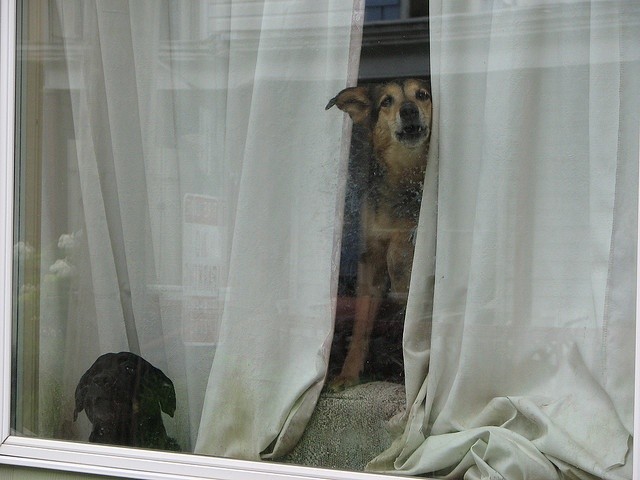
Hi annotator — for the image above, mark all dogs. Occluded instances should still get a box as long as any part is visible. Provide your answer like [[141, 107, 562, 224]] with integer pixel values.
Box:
[[73, 352, 182, 452], [325, 76, 432, 393]]
